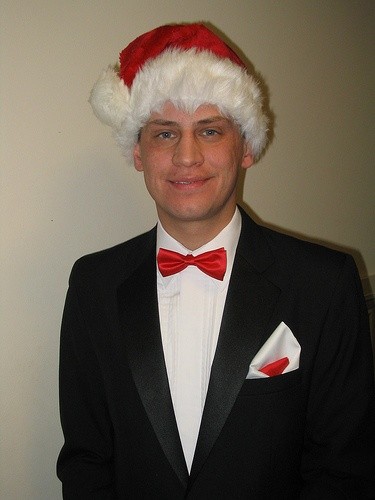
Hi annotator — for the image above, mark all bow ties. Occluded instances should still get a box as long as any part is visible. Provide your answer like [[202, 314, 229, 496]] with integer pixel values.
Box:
[[157, 247, 227, 281]]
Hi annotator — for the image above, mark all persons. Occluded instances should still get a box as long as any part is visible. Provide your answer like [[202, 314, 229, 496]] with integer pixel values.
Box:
[[56, 22, 374, 500]]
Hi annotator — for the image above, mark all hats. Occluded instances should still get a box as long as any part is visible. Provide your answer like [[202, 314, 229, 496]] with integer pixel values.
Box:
[[90, 23, 269, 161]]
